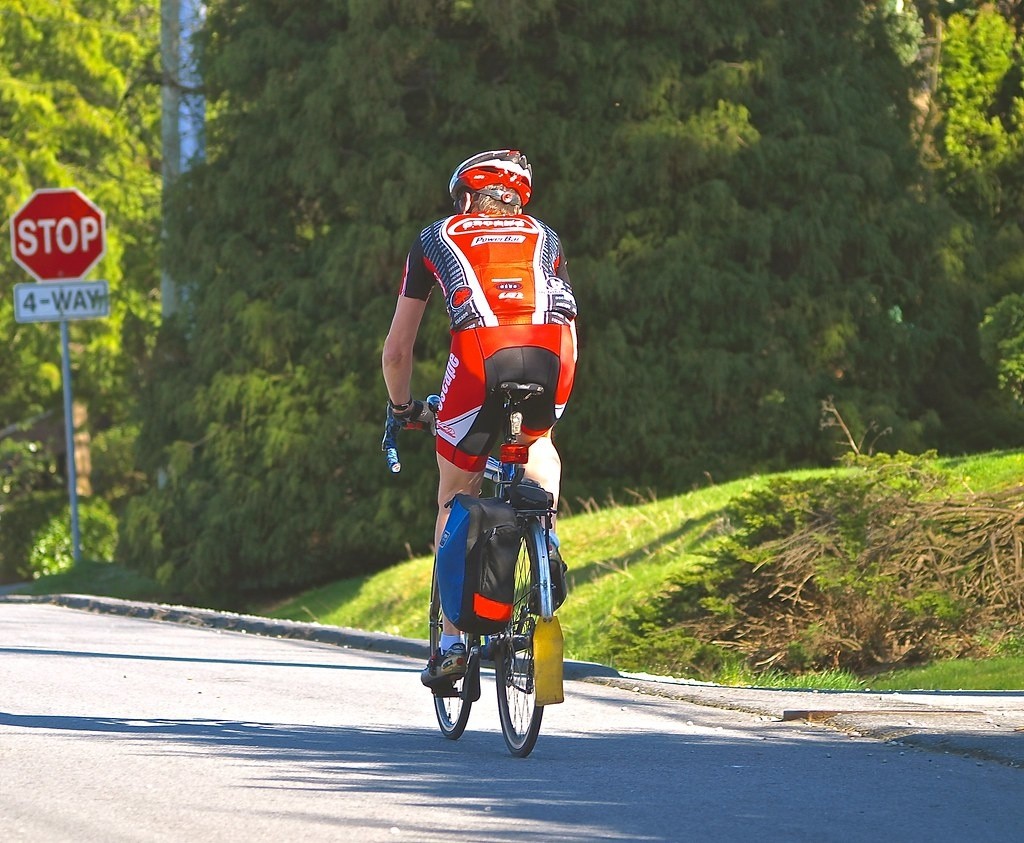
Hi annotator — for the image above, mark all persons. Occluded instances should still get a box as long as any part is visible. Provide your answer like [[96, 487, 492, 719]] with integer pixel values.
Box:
[[381, 150, 578, 684]]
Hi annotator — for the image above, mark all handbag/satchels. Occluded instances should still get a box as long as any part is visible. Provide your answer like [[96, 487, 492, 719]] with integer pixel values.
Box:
[[438, 490, 521, 636]]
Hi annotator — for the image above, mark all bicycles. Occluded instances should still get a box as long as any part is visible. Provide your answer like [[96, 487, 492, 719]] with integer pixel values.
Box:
[[384, 382, 565, 757]]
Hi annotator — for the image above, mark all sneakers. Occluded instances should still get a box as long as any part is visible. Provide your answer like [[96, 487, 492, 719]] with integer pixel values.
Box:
[[527, 534, 567, 616], [421, 643, 468, 688]]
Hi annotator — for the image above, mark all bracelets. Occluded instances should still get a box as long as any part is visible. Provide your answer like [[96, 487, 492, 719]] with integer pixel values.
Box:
[[389, 394, 412, 411]]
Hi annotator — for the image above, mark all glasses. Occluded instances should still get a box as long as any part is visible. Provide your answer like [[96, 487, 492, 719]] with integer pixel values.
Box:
[[454, 191, 467, 214]]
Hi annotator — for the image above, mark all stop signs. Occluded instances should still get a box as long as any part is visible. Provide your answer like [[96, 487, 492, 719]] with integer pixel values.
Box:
[[10, 188, 106, 281]]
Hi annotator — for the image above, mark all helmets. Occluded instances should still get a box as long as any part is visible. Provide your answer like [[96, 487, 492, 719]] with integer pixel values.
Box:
[[449, 149, 533, 208]]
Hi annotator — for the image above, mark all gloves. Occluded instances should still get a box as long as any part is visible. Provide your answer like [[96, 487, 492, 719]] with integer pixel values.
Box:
[[394, 400, 436, 438]]
